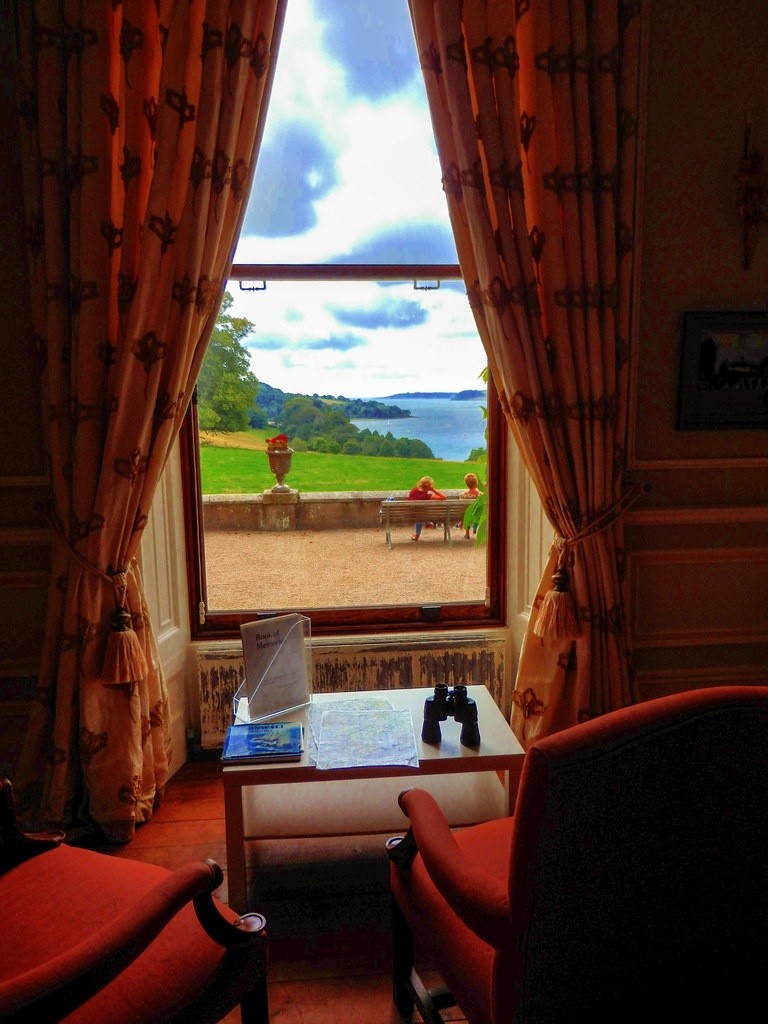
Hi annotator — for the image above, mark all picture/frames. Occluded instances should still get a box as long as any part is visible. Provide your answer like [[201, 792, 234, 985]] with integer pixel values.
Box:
[[676, 309, 768, 433]]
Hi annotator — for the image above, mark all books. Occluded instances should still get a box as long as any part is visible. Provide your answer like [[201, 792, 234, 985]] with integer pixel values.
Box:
[[241, 613, 310, 718], [221, 722, 304, 764]]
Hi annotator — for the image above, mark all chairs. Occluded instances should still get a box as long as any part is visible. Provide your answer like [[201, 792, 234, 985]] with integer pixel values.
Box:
[[384, 684, 768, 1024], [0, 771, 270, 1024]]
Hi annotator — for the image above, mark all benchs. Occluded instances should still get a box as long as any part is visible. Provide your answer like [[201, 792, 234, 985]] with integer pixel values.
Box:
[[379, 498, 487, 550]]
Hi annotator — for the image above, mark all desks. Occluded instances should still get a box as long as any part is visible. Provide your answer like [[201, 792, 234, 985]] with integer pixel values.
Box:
[[223, 683, 526, 914]]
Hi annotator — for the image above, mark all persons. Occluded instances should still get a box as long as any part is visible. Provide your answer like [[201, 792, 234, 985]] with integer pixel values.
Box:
[[408, 476, 447, 540], [457, 473, 484, 539]]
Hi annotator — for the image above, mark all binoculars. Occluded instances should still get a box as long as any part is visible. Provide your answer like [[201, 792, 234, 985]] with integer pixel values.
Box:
[[420, 683, 481, 747]]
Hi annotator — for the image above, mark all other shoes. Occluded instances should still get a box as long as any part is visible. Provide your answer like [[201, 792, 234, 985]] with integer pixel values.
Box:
[[426, 522, 434, 529], [455, 522, 463, 528], [464, 534, 470, 539], [411, 533, 419, 541]]
